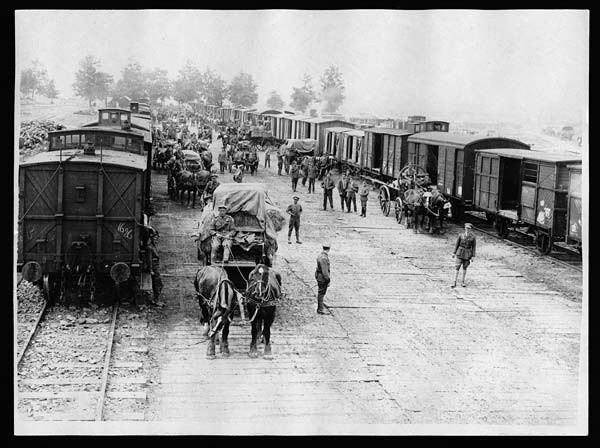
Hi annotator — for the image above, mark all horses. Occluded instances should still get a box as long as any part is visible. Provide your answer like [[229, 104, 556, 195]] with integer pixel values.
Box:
[[428, 188, 453, 234], [193, 263, 238, 361], [244, 261, 282, 361], [401, 187, 432, 235]]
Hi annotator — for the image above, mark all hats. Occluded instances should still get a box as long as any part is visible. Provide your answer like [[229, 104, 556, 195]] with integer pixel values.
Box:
[[294, 196, 299, 200], [323, 244, 331, 248], [465, 223, 473, 229]]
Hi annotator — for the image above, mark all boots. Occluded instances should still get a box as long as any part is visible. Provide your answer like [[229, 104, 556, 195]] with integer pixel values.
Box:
[[360, 206, 366, 217]]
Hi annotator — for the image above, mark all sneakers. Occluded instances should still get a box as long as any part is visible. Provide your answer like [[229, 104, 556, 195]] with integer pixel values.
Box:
[[318, 309, 326, 315], [288, 241, 292, 244], [296, 240, 302, 244], [451, 284, 456, 287], [461, 283, 466, 287]]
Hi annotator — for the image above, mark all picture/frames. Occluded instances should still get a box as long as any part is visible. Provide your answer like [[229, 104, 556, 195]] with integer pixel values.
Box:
[[0, 0, 600, 448]]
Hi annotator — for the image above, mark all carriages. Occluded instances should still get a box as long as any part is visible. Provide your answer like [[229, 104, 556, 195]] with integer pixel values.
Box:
[[377, 160, 452, 236], [217, 139, 260, 176], [278, 136, 338, 181], [166, 149, 219, 210], [191, 181, 287, 361]]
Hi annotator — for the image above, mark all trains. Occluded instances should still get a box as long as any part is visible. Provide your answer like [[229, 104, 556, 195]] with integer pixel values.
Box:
[[18, 96, 165, 307], [185, 98, 583, 257]]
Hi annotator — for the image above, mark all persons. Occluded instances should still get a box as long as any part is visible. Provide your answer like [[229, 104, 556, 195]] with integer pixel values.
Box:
[[320, 172, 335, 212], [313, 242, 335, 317], [308, 161, 318, 194], [174, 147, 186, 171], [210, 205, 235, 264], [300, 157, 308, 186], [286, 196, 303, 246], [346, 177, 358, 214], [359, 181, 369, 217], [290, 160, 300, 192], [146, 230, 166, 307], [264, 146, 271, 168], [218, 146, 227, 173], [204, 174, 220, 194], [235, 164, 244, 184], [452, 223, 476, 290], [276, 151, 285, 176], [337, 172, 349, 212]]
